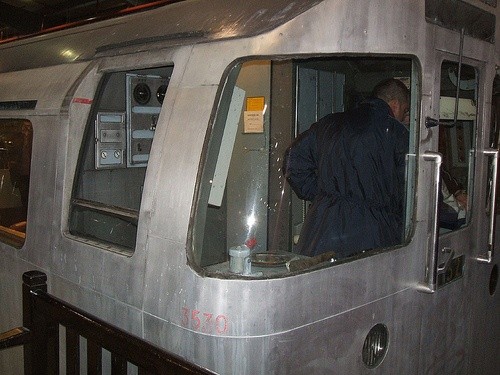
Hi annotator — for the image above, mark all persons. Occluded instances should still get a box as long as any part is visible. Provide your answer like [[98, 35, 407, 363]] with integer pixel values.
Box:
[[8, 120, 32, 211], [282, 78, 471, 262]]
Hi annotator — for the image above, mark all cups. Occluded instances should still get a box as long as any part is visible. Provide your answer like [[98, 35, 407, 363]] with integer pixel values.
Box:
[[229, 246, 250, 273]]
